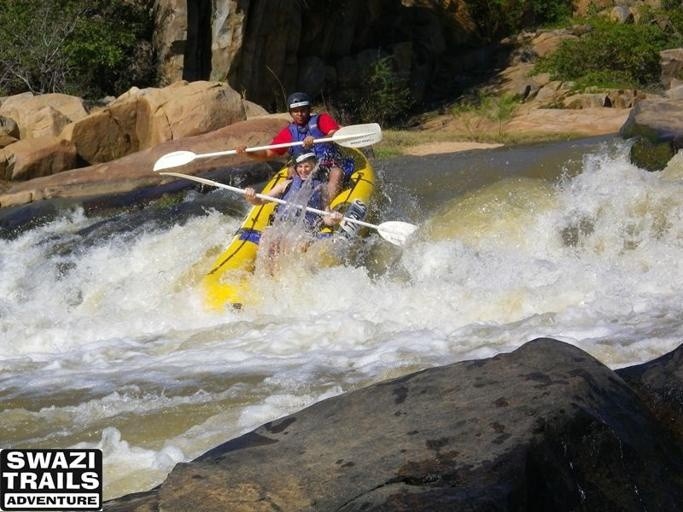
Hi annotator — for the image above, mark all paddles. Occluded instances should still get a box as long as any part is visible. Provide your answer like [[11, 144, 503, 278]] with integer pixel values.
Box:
[[159, 171, 419, 245], [153, 122, 383, 172]]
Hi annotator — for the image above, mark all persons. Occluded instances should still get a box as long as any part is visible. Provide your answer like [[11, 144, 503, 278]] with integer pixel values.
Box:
[[231, 91, 354, 207], [242, 147, 345, 280]]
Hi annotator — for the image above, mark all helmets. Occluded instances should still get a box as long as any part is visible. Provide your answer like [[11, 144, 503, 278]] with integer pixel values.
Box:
[[287, 92, 312, 113], [292, 150, 316, 166]]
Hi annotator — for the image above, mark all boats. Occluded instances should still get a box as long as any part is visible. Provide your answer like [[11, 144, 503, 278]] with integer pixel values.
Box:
[[171, 140, 379, 316]]
